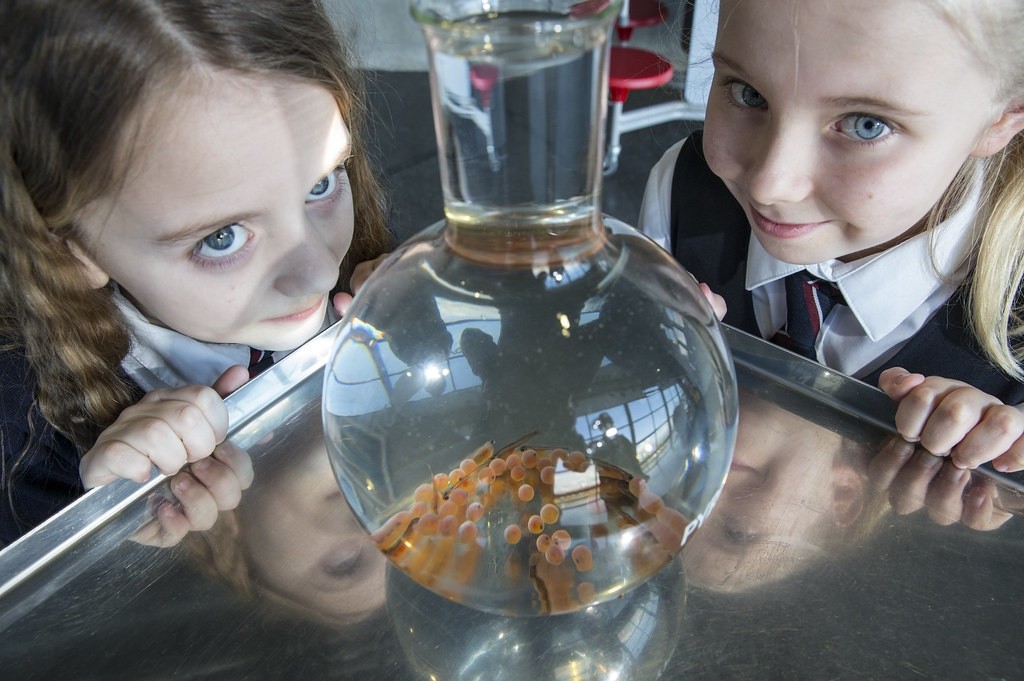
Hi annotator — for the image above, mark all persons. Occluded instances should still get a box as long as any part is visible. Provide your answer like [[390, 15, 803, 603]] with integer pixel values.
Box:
[[638, 0, 1024, 473], [677, 390, 1013, 591], [132, 412, 385, 625], [0, 0, 403, 552]]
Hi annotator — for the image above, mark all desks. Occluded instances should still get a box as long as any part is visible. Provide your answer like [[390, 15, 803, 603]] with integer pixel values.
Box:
[[0, 315, 1024, 681]]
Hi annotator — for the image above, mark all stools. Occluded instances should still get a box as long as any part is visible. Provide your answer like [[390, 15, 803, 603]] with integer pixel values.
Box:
[[601, 46, 674, 176], [615, 0, 668, 48]]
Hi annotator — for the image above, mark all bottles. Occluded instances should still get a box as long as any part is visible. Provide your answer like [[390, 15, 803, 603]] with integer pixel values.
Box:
[[320, 0, 742, 620]]
